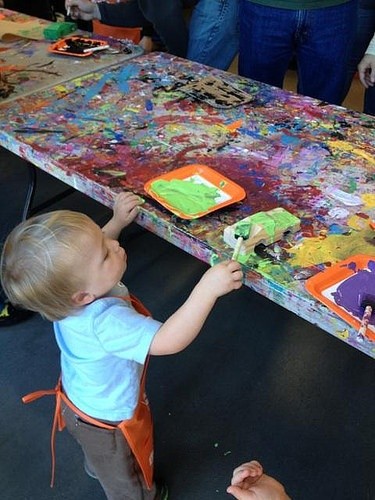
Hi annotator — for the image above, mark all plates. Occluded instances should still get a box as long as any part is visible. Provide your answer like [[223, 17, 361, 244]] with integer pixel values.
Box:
[[179, 76, 255, 109], [48, 34, 107, 56], [143, 163, 246, 219], [304, 251, 375, 343]]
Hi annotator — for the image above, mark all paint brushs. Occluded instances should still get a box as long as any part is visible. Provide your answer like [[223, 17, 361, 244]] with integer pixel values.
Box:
[[67, 0, 71, 16]]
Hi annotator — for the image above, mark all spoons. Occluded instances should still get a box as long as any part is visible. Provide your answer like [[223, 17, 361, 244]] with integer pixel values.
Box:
[[230, 219, 250, 261]]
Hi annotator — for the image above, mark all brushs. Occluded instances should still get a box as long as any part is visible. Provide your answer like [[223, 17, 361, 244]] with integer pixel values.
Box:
[[356, 293, 375, 342], [231, 221, 251, 262]]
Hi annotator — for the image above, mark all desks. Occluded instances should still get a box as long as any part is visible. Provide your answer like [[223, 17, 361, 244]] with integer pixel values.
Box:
[[0, 8, 143, 121], [0, 51, 375, 363]]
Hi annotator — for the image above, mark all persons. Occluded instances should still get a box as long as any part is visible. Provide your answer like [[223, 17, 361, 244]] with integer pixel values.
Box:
[[0, 192, 243, 500], [0, 0, 375, 117], [227, 460, 291, 500]]
